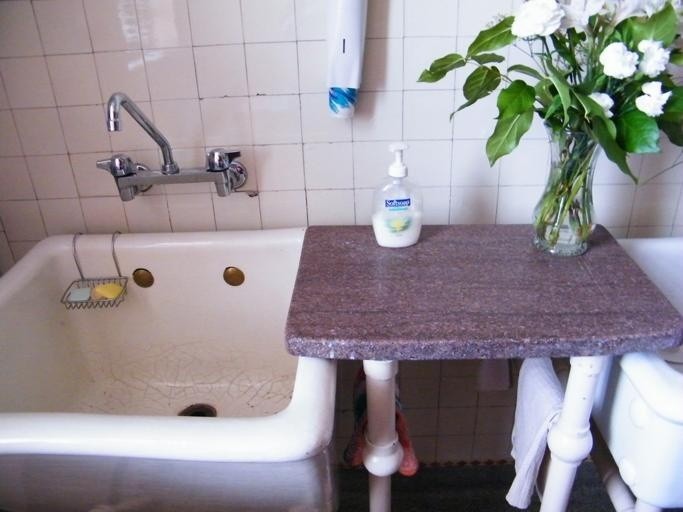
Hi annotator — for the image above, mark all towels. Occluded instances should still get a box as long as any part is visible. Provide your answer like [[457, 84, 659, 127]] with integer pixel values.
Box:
[[502, 357, 566, 509], [342, 366, 420, 478]]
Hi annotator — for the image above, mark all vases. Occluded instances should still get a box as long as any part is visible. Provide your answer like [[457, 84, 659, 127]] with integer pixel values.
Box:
[[532, 124, 597, 259]]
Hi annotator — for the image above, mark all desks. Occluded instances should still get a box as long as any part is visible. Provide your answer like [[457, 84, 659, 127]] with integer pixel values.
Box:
[[282, 223, 683, 512]]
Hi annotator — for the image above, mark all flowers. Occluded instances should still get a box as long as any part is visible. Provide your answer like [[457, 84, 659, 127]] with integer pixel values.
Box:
[[417, 0, 682, 247]]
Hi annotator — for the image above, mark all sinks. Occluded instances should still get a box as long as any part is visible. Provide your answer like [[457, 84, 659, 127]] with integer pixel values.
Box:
[[590, 237, 683, 511], [0, 223, 342, 512]]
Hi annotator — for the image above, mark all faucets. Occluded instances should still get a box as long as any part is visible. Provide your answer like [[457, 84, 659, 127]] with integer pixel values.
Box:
[[108, 91, 178, 176]]
